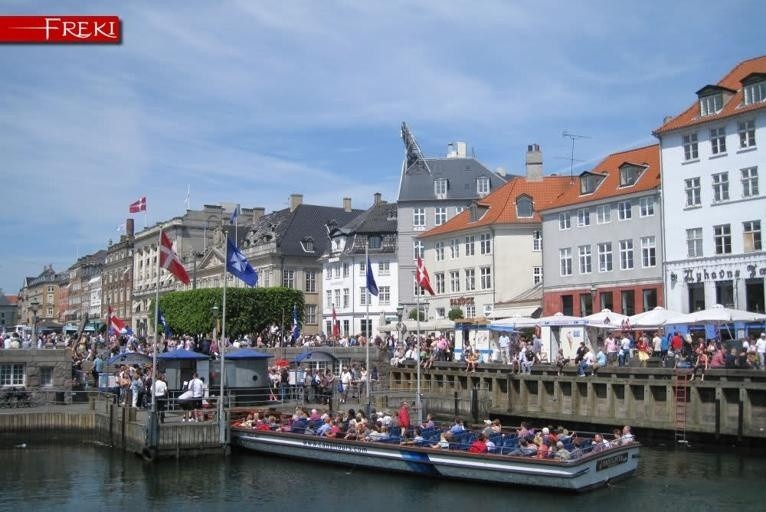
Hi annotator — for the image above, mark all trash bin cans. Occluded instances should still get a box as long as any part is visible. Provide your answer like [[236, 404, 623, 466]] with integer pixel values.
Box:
[[55, 392, 64, 404], [676, 362, 691, 368]]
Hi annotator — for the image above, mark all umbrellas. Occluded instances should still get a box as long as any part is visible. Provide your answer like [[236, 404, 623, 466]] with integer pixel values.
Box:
[[619, 304, 698, 338], [682, 302, 766, 343], [581, 308, 631, 331], [487, 314, 542, 332], [538, 310, 587, 330]]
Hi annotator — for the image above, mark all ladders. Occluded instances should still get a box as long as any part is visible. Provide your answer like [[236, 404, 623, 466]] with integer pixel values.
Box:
[[674, 374, 687, 441]]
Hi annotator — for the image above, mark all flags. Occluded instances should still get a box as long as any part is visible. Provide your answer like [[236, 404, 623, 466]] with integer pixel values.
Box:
[[330, 303, 341, 342], [157, 223, 192, 288], [414, 241, 436, 298], [224, 228, 259, 288], [106, 295, 136, 340], [156, 305, 174, 338], [292, 300, 302, 343], [128, 196, 150, 214], [364, 239, 380, 299]]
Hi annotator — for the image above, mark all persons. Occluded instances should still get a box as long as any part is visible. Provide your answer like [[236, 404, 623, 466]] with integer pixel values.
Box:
[[0, 320, 766, 464]]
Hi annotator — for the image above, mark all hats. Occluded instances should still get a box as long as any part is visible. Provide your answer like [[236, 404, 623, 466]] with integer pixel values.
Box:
[[375, 408, 394, 417], [483, 419, 491, 425]]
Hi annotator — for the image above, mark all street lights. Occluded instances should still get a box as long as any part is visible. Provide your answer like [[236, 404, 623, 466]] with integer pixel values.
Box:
[[31, 297, 39, 348], [396, 305, 404, 345], [211, 303, 219, 349]]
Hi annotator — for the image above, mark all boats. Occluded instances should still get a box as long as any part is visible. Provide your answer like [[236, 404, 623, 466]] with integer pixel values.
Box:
[[230, 422, 641, 489]]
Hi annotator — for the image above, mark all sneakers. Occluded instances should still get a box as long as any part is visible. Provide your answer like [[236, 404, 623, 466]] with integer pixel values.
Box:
[[182, 418, 193, 422]]
